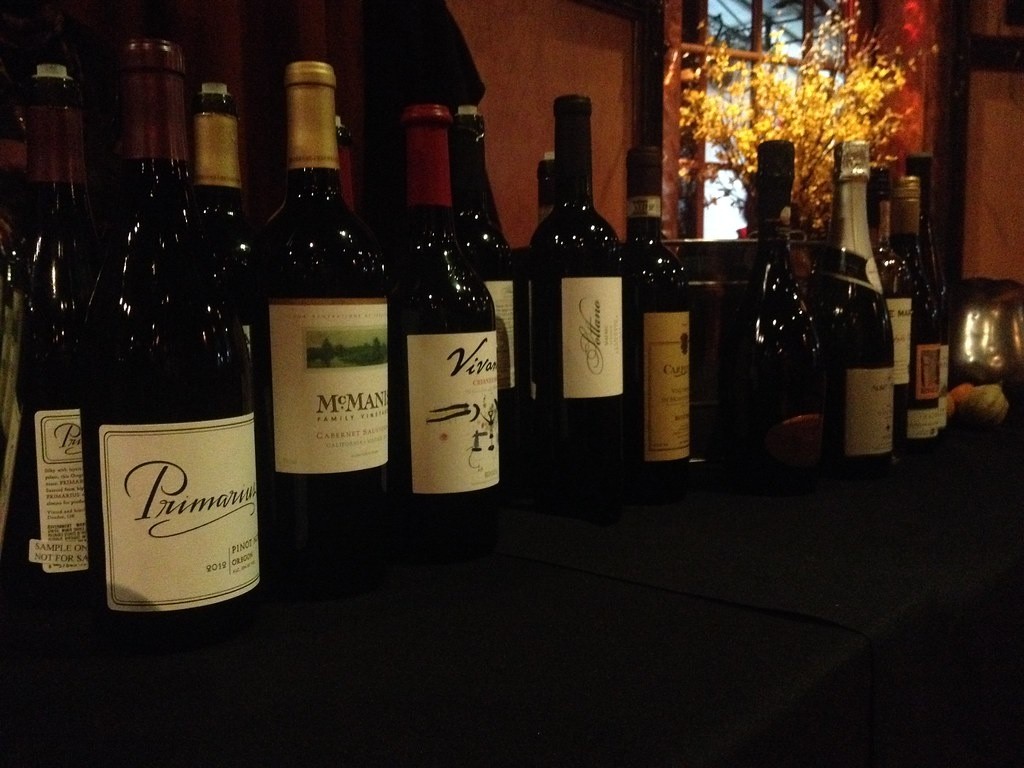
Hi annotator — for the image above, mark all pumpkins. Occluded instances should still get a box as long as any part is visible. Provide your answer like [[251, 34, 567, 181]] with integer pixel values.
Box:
[[960, 383, 1008, 425]]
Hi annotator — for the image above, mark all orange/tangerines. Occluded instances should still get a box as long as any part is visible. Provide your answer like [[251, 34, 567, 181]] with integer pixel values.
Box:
[[951, 386, 974, 406]]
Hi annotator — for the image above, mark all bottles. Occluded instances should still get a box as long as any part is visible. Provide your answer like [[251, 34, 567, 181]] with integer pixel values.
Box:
[[714, 139, 826, 496], [610, 144, 694, 499], [876, 174, 950, 455], [525, 95, 623, 507], [0, 62, 107, 614], [808, 137, 895, 481], [385, 102, 524, 565], [245, 58, 387, 603], [88, 36, 266, 657]]
